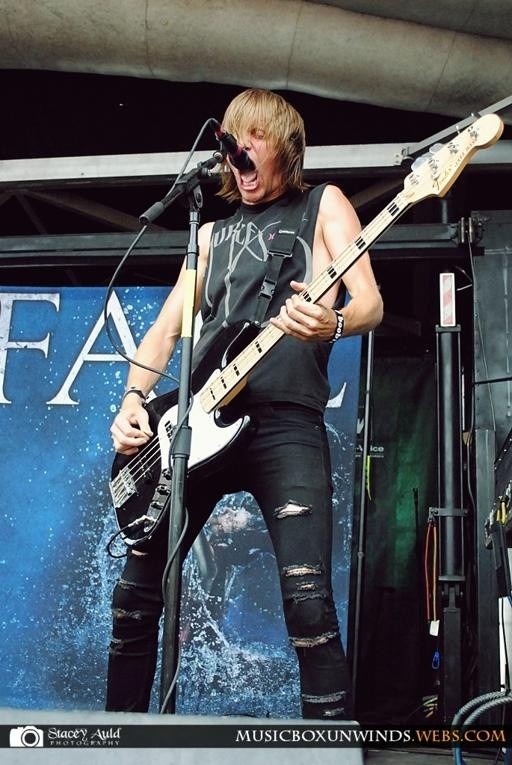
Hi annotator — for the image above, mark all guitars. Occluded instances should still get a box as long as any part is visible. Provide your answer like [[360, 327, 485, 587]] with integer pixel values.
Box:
[[112, 115, 504, 553]]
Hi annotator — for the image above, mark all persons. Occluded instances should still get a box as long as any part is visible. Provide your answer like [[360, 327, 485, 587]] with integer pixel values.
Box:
[[105, 85, 385, 720]]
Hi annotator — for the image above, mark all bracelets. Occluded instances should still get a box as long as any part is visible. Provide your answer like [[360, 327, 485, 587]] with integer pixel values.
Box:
[[321, 307, 344, 344], [122, 386, 146, 403]]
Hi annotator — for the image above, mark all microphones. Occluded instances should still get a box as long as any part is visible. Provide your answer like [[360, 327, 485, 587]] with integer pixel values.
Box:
[[208, 118, 251, 171]]
